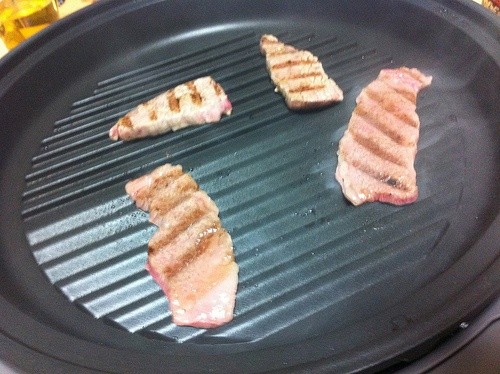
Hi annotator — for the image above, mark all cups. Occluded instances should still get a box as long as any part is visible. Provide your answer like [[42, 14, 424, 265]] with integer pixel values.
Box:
[[2, 0, 56, 51]]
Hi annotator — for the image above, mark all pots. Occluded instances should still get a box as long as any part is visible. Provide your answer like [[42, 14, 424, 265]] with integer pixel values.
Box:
[[1, 0, 500, 374]]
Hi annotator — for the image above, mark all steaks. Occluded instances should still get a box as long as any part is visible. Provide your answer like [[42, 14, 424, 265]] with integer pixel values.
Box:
[[121, 164, 239, 327], [257, 33, 344, 109], [107, 77, 235, 142], [333, 67, 433, 209]]
